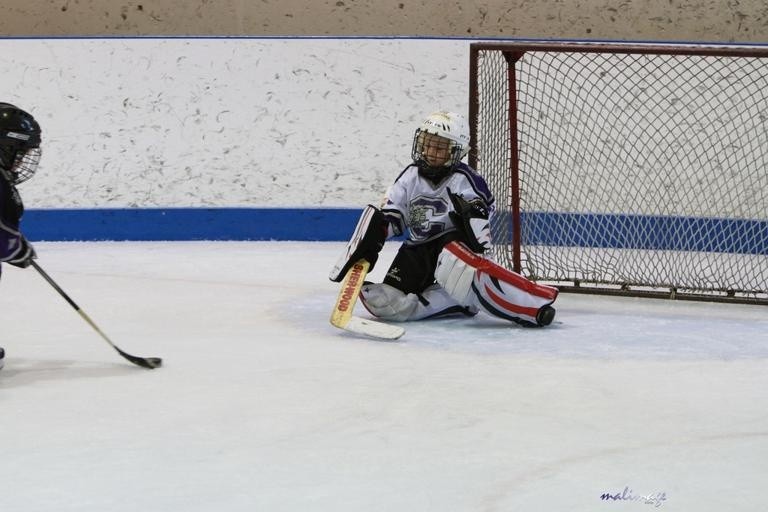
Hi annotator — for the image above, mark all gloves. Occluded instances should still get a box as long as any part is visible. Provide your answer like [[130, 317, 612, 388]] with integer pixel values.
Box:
[[5, 234, 38, 269]]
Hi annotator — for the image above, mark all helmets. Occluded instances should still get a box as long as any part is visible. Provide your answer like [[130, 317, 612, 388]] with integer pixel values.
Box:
[[411, 111, 471, 185], [0, 101, 42, 187]]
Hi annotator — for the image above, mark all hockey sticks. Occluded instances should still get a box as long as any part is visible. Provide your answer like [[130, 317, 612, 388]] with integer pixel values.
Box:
[[330, 259, 405, 340], [30, 260, 161, 369]]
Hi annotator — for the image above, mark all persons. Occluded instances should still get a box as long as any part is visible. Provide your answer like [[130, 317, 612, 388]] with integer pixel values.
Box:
[[329, 109, 559, 328], [0, 103, 42, 368]]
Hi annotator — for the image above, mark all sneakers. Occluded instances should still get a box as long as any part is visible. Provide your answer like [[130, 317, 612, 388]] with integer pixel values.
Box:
[[540, 305, 555, 325]]
